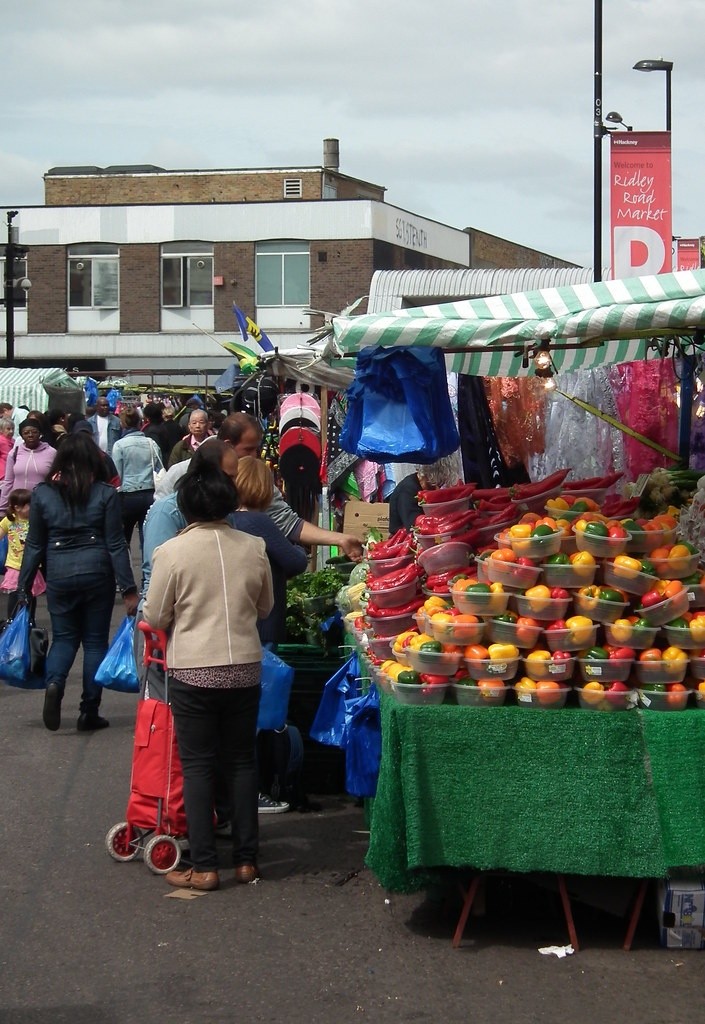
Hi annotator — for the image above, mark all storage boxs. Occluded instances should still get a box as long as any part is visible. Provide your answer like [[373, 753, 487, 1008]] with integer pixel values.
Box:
[[656, 868, 705, 950]]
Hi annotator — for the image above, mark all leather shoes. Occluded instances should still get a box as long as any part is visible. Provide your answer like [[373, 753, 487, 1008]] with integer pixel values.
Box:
[[166, 867, 219, 889], [235, 862, 260, 883]]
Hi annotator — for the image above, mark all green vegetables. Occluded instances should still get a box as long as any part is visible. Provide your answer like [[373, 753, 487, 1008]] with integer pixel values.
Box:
[[287, 567, 344, 636]]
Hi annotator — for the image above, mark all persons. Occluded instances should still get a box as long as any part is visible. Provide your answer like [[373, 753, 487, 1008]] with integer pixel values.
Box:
[[0, 488, 47, 628], [389, 453, 464, 533], [0, 394, 368, 891], [17, 432, 140, 731], [0, 418, 58, 518], [141, 464, 275, 892]]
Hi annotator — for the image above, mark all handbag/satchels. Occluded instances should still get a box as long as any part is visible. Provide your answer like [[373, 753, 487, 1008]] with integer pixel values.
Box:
[[0, 599, 32, 686], [257, 647, 294, 731], [8, 597, 49, 688], [94, 613, 141, 693], [147, 438, 167, 501]]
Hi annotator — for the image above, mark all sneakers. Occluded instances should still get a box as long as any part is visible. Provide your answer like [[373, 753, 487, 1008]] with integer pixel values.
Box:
[[256, 791, 291, 814], [214, 821, 232, 838]]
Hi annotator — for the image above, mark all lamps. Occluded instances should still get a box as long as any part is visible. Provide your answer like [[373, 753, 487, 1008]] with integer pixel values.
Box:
[[529, 339, 559, 379]]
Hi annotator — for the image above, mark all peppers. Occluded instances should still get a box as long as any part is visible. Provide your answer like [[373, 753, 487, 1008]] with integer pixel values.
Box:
[[413, 468, 626, 510], [353, 495, 705, 707]]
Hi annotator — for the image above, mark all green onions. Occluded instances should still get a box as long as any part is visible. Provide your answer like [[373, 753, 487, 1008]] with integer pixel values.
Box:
[[643, 468, 705, 503]]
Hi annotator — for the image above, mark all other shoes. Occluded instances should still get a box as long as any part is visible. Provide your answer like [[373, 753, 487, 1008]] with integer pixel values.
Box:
[[76, 708, 109, 730], [42, 680, 61, 731]]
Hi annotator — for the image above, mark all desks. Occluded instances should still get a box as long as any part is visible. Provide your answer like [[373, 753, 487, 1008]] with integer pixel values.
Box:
[[326, 619, 705, 953]]
